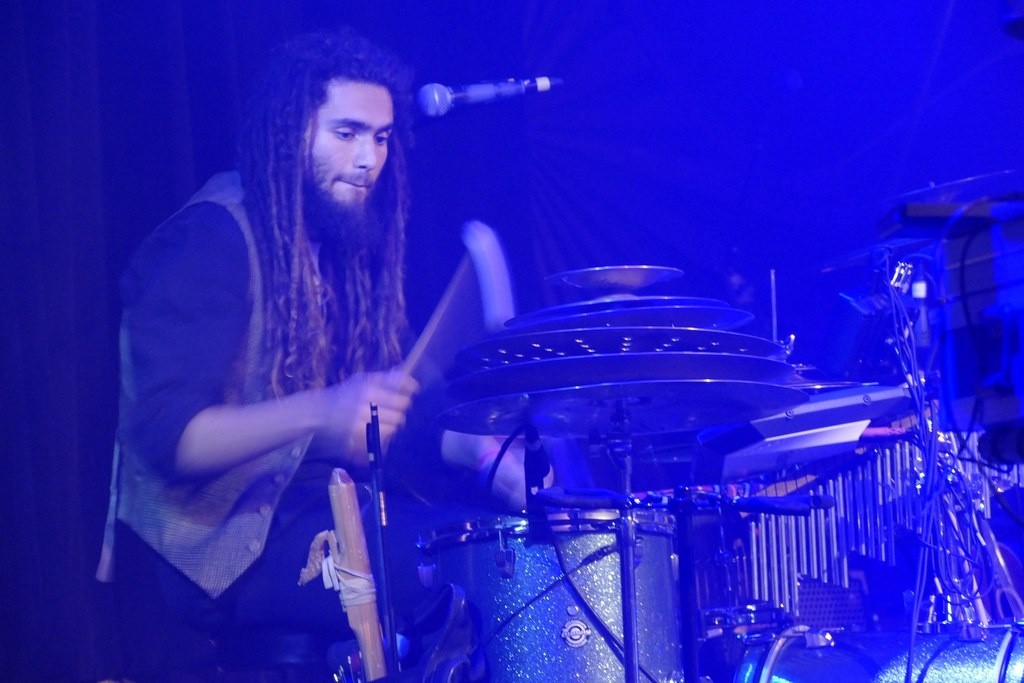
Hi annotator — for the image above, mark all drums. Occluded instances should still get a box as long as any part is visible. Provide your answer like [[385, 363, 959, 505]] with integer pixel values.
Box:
[[418, 510, 676, 683]]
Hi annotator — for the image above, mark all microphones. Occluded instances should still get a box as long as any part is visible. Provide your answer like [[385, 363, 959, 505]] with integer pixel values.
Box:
[[523, 427, 553, 492], [416, 77, 563, 115]]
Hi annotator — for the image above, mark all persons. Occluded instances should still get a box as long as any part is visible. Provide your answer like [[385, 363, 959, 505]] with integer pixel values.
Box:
[[102, 27, 554, 682]]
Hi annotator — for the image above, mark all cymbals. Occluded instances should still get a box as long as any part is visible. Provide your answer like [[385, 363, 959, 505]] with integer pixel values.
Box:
[[438, 263, 879, 422]]
